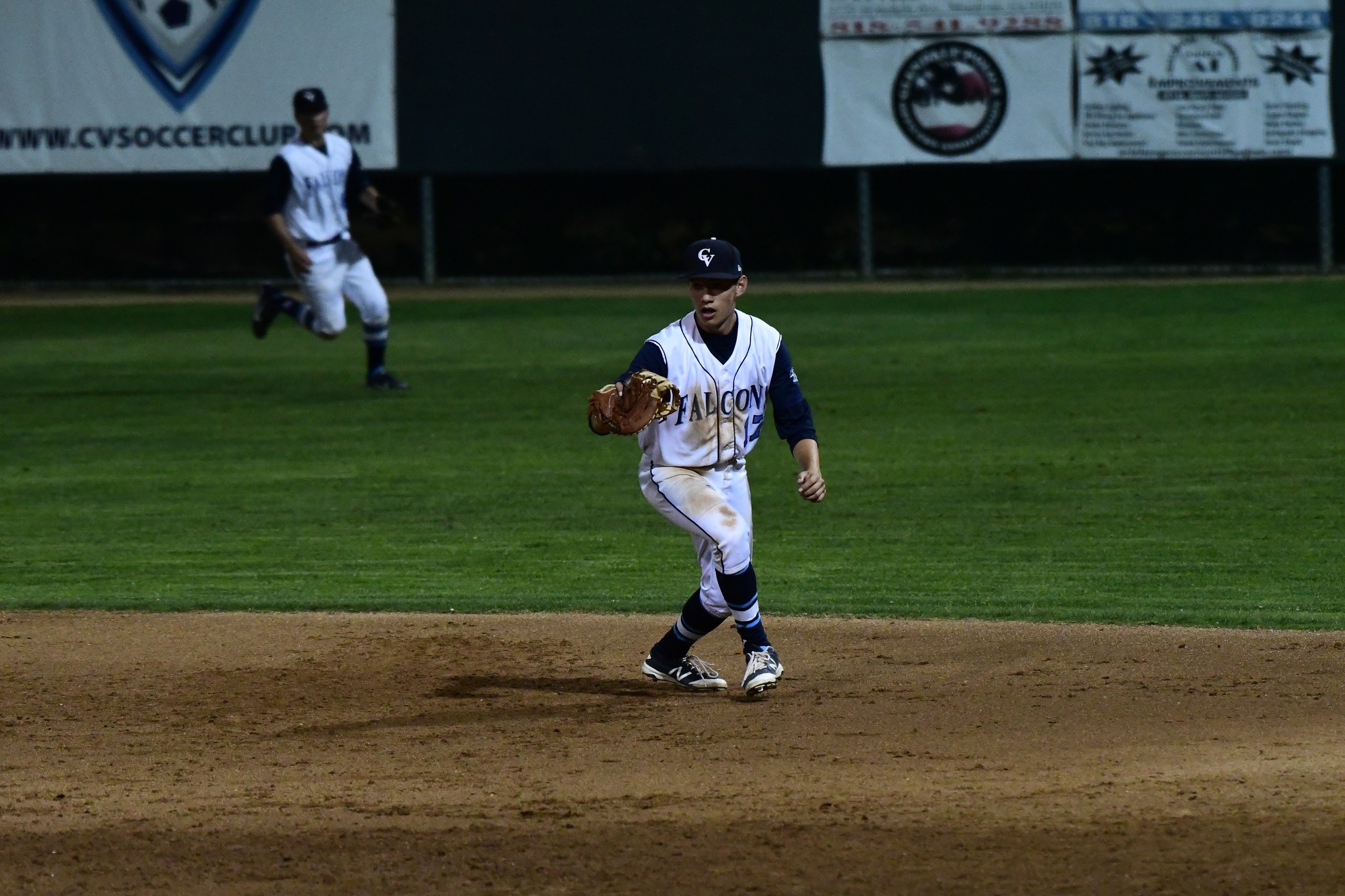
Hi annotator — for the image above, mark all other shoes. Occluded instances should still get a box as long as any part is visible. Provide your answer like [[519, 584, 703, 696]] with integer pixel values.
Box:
[[368, 373, 408, 390], [252, 286, 280, 336]]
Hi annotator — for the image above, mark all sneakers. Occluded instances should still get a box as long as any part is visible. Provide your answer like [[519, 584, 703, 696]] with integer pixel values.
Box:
[[740, 644, 784, 694], [643, 644, 728, 691]]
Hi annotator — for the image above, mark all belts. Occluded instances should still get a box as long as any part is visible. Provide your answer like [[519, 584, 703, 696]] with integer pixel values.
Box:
[[309, 235, 341, 246]]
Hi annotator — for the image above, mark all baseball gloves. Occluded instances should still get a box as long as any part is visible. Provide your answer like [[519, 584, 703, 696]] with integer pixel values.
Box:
[[587, 371, 682, 440]]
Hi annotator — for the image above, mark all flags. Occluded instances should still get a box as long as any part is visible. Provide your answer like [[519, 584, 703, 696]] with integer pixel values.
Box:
[[0, 0, 396, 171], [821, 0, 1335, 167]]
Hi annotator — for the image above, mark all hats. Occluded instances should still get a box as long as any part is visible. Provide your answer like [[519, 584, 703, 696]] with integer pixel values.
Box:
[[294, 89, 325, 116], [678, 237, 741, 279]]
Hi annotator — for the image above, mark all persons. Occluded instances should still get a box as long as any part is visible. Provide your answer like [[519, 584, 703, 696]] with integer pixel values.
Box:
[[588, 235, 827, 696], [254, 89, 402, 393]]
